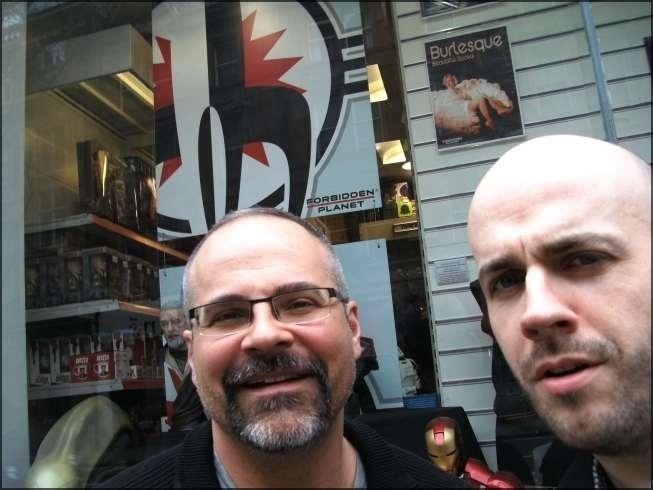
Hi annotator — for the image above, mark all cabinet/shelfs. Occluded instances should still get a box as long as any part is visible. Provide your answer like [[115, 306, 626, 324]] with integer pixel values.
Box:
[[25, 214, 188, 400]]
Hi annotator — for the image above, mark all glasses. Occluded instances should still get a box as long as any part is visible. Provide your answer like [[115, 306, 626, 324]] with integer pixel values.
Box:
[[189, 288, 344, 337]]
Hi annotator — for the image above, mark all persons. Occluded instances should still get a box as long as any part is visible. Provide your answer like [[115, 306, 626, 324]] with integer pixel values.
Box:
[[441, 71, 514, 131], [159, 300, 208, 432], [97, 207, 479, 490], [467, 134, 653, 489]]
